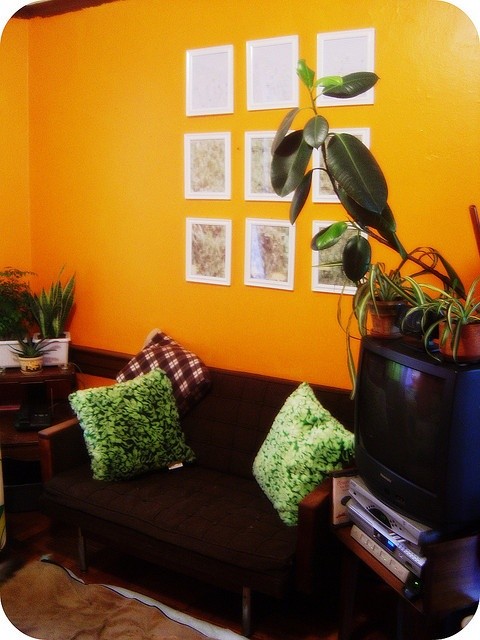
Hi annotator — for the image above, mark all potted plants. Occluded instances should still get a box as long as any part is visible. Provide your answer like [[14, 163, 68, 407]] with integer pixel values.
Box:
[[400, 274, 480, 371], [5, 327, 60, 376], [31, 265, 76, 367], [0, 266, 38, 369], [311, 246, 440, 401]]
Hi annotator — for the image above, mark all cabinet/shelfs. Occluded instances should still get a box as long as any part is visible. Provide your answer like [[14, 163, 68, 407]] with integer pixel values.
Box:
[[326, 467, 480, 640]]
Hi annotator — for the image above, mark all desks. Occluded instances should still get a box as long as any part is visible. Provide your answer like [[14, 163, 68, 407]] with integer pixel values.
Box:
[[0, 362, 82, 511]]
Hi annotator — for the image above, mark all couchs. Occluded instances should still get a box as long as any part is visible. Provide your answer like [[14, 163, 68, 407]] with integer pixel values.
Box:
[[37, 366, 355, 640]]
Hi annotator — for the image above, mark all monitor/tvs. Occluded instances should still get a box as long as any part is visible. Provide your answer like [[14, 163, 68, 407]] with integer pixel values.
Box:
[[354, 334, 480, 538]]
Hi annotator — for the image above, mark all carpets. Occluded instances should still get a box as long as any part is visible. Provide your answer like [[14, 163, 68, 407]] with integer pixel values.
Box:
[[0, 552, 254, 640]]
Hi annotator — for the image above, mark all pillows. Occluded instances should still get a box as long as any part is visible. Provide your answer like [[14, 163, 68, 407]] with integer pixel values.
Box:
[[249, 379, 356, 528], [112, 330, 213, 419], [68, 367, 200, 481]]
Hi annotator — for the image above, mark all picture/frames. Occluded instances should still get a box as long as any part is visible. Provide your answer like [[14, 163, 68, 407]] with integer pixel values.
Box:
[[184, 131, 232, 200], [244, 130, 296, 202], [312, 127, 371, 205], [246, 34, 299, 111], [185, 217, 232, 286], [311, 220, 368, 296], [186, 44, 234, 117], [244, 217, 295, 291], [316, 28, 375, 107]]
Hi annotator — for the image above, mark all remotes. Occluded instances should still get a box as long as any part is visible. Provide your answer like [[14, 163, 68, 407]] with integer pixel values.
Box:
[[404, 578, 422, 601]]
